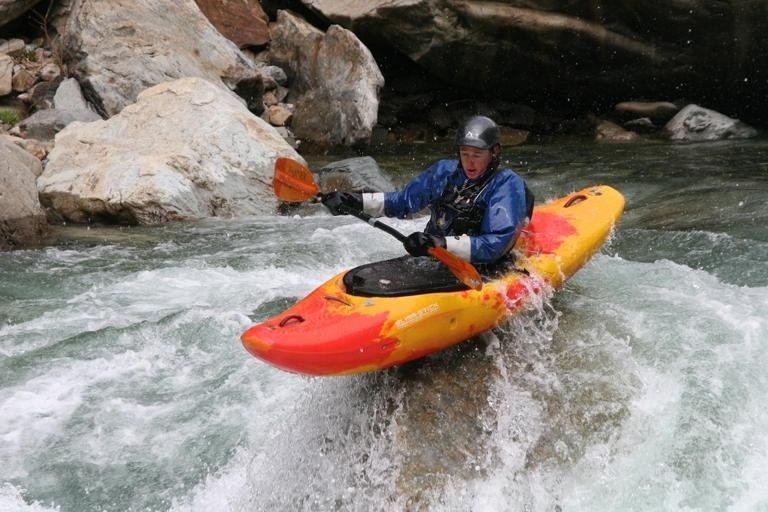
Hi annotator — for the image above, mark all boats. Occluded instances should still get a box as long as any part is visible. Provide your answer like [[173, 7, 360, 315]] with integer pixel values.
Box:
[[241, 184, 626, 377]]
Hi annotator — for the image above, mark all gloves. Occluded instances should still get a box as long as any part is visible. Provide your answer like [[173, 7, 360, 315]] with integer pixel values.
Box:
[[404, 232, 447, 259], [321, 188, 363, 217]]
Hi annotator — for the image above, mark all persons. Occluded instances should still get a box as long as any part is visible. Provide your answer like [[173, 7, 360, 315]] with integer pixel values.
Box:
[[321, 114, 538, 265]]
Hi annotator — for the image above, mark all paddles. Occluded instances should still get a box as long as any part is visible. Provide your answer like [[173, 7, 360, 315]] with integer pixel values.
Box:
[[273, 158, 482, 291]]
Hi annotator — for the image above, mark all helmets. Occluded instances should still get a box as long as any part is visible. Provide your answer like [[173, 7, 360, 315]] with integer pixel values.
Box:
[[455, 116, 500, 149]]
